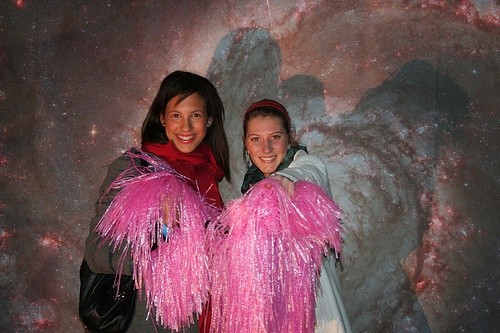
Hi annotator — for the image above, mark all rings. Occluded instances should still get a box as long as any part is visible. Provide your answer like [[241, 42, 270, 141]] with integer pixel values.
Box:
[[271, 173, 276, 176]]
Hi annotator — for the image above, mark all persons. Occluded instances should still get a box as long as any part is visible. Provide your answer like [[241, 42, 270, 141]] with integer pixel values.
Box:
[[83, 70, 231, 333], [241, 98, 352, 333]]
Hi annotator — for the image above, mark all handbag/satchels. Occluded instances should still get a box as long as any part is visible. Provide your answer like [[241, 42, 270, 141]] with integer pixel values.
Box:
[[79, 259, 138, 333]]
